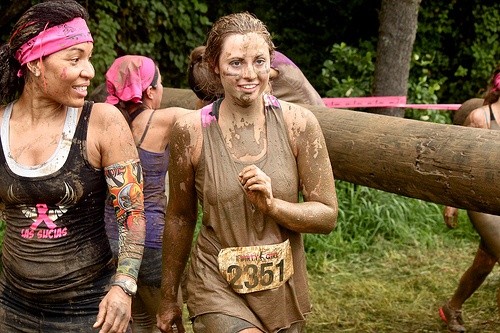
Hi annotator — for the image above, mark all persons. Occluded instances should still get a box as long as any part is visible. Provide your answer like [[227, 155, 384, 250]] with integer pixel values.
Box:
[[187, 45, 325, 106], [105, 54, 198, 333], [439, 72, 500, 333], [155, 13, 337, 333], [0, 0, 145, 333]]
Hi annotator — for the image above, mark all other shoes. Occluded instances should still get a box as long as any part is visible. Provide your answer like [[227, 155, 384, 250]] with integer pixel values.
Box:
[[439, 302, 469, 332]]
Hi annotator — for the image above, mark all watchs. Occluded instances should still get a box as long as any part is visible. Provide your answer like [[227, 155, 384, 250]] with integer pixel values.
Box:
[[112, 279, 138, 296]]
